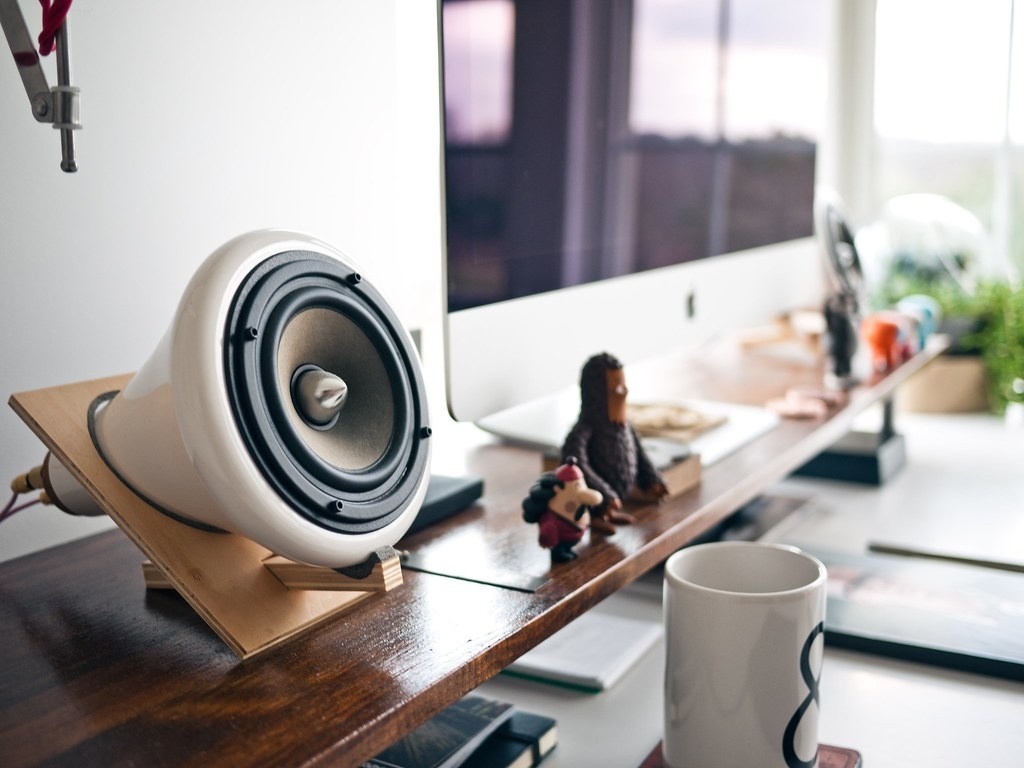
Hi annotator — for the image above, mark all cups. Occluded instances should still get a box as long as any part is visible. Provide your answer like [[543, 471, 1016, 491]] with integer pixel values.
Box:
[[661, 540, 828, 768]]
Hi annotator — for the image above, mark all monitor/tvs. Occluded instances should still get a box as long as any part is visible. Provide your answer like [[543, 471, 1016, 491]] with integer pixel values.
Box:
[[437, 0, 833, 469]]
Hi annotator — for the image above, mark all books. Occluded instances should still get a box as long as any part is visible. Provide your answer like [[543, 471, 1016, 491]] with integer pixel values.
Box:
[[360, 695, 559, 768], [503, 613, 663, 690], [819, 548, 1024, 678]]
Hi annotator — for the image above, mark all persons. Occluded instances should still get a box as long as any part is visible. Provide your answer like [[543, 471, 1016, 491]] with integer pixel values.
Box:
[[523, 459, 602, 565], [560, 353, 671, 526]]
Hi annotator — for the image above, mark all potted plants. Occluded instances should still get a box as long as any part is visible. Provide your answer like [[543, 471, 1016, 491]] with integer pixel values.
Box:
[[873, 252, 1023, 415]]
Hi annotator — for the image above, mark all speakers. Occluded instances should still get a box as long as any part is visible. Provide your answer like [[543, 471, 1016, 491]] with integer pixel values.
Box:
[[44, 227, 431, 568]]
